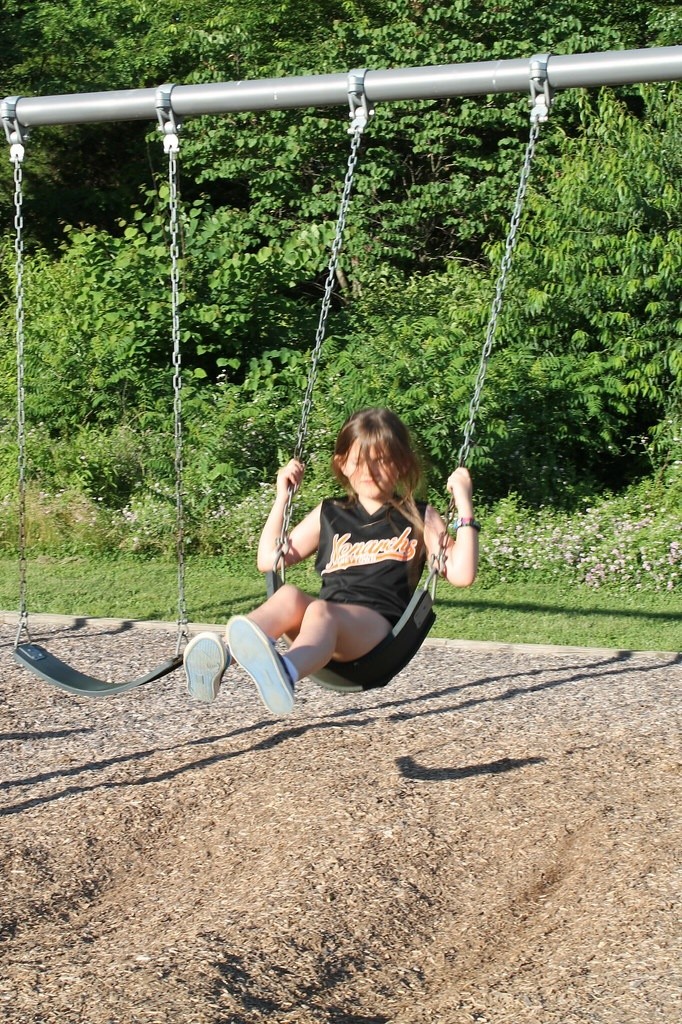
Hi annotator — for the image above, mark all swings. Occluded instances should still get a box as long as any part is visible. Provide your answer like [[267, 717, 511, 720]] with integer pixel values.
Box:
[[6, 109, 193, 698], [266, 76, 552, 693]]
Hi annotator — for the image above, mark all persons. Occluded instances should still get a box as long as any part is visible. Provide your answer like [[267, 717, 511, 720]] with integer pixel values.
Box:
[[183, 408, 479, 715]]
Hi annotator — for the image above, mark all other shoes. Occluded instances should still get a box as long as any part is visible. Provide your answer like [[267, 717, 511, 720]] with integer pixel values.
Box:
[[183, 632, 229, 703], [226, 615, 294, 714]]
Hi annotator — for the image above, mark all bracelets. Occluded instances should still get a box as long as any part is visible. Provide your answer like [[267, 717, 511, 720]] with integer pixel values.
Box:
[[454, 518, 482, 532]]
